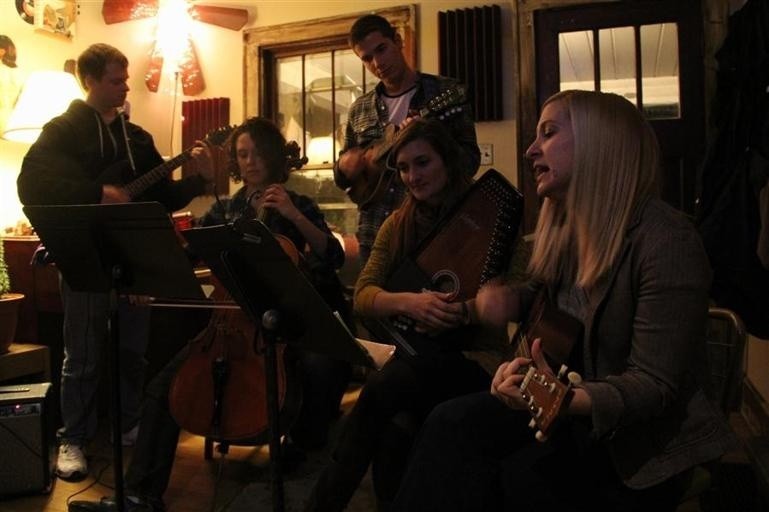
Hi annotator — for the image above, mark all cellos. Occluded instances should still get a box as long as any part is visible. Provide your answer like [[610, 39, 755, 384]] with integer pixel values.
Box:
[[169, 140, 308, 446]]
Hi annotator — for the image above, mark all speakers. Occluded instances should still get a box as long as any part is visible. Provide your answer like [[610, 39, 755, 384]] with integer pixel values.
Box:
[[0, 381, 58, 502]]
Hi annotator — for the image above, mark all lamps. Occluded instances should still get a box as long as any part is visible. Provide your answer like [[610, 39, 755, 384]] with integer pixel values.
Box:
[[103, 0, 247, 95]]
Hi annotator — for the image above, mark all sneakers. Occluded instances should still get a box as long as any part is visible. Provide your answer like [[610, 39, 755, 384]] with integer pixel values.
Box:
[[67, 495, 157, 512], [53, 443, 88, 481], [109, 427, 138, 446], [275, 437, 299, 470]]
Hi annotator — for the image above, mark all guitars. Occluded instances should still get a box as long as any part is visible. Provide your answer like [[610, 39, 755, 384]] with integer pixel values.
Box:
[[507, 287, 582, 442], [345, 85, 466, 205], [124, 124, 236, 198]]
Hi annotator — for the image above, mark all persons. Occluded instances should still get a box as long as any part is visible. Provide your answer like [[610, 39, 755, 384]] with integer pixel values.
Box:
[[98, 115, 347, 511], [386, 90, 743, 511], [15, 42, 217, 482], [330, 11, 482, 346], [298, 117, 534, 509]]
